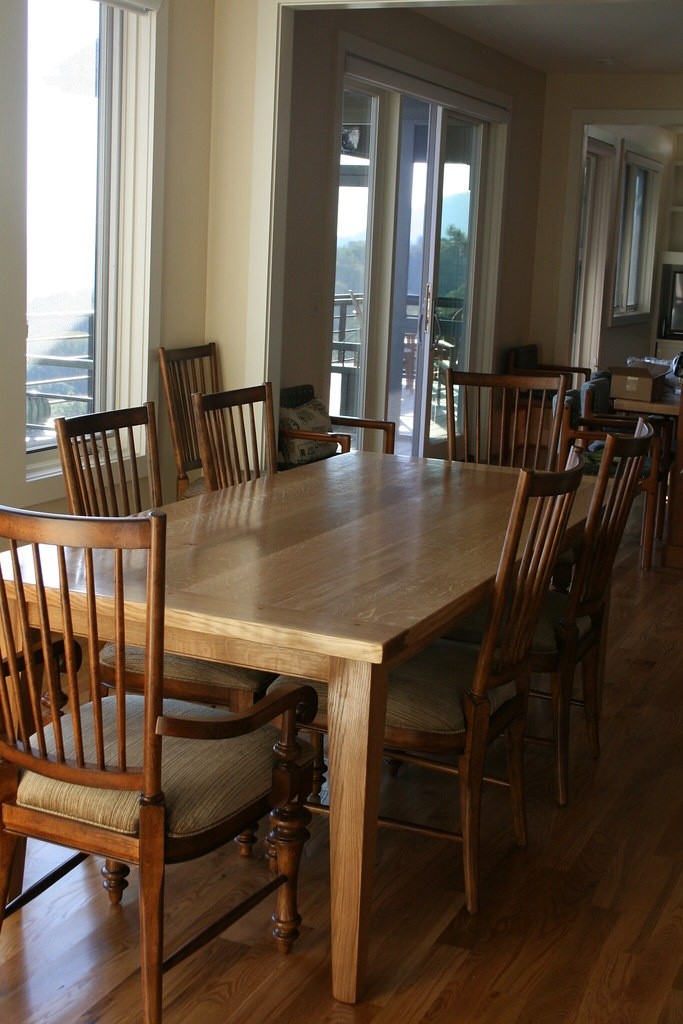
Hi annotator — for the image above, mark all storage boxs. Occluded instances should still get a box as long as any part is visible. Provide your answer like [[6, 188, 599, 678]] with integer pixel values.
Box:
[[607, 361, 672, 402]]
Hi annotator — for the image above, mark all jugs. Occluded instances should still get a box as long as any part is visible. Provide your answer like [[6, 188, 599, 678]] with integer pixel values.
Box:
[[672, 351, 683, 376]]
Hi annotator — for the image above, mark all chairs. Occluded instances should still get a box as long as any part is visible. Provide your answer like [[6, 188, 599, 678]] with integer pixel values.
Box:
[[0, 505, 318, 1024], [55, 341, 682, 958]]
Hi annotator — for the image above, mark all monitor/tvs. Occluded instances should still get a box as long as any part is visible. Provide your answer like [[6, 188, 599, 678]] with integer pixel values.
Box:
[[656, 264, 683, 340]]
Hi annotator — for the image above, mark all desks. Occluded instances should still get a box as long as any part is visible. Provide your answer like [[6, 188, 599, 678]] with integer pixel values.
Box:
[[0, 448, 617, 1000], [614, 372, 683, 567]]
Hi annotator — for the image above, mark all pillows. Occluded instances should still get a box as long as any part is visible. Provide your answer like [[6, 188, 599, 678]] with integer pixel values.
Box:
[[280, 399, 337, 465]]
